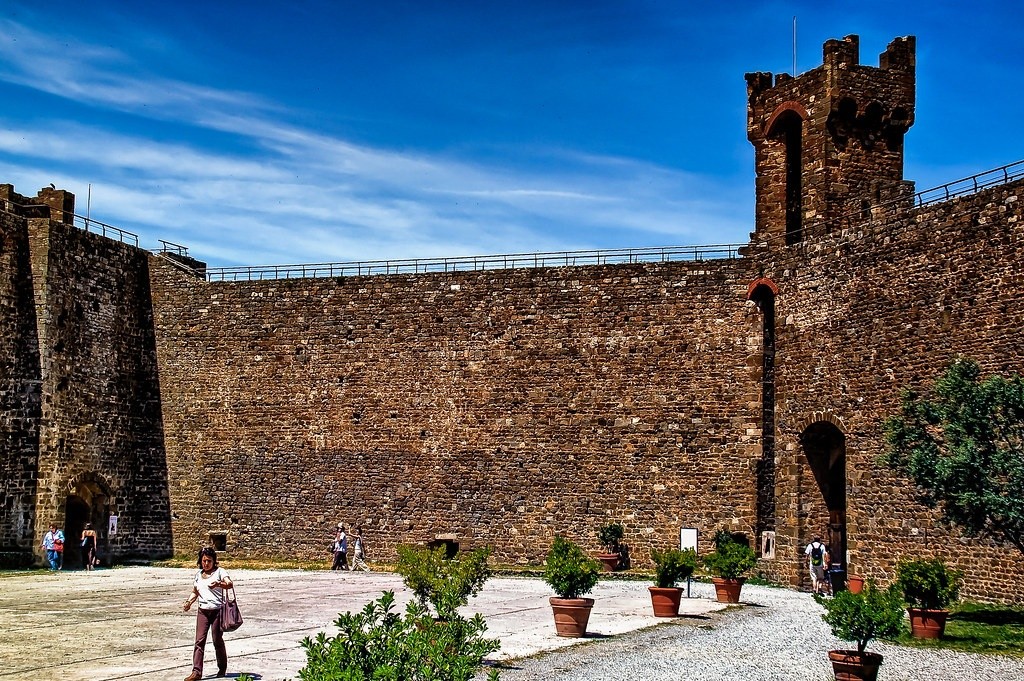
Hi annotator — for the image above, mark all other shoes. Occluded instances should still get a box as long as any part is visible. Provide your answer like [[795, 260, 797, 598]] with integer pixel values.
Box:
[[184, 672, 202, 681], [217, 669, 226, 677]]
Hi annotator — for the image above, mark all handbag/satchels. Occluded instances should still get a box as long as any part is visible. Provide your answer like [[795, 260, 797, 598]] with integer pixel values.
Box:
[[80, 537, 88, 546], [327, 542, 335, 554], [53, 542, 63, 552], [361, 546, 366, 558], [219, 582, 243, 631]]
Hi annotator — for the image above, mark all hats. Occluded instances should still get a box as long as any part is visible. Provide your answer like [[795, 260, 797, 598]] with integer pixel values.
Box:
[[338, 522, 344, 528]]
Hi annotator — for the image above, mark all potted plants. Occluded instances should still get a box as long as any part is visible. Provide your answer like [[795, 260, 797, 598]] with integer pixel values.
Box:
[[648, 545, 696, 618], [811, 574, 911, 681], [597, 524, 624, 573], [702, 539, 757, 605], [389, 540, 491, 655], [542, 534, 600, 637], [896, 557, 964, 640]]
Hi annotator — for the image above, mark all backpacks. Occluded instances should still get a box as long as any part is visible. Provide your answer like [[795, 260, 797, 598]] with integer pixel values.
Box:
[[810, 543, 824, 566]]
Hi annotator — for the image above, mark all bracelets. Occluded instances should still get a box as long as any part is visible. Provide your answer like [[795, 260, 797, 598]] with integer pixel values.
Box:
[[186, 601, 190, 605]]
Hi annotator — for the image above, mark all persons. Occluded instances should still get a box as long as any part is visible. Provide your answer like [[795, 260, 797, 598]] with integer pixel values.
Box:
[[806, 536, 845, 596], [80, 523, 98, 571], [183, 548, 232, 681], [330, 525, 370, 572], [43, 524, 65, 572]]
